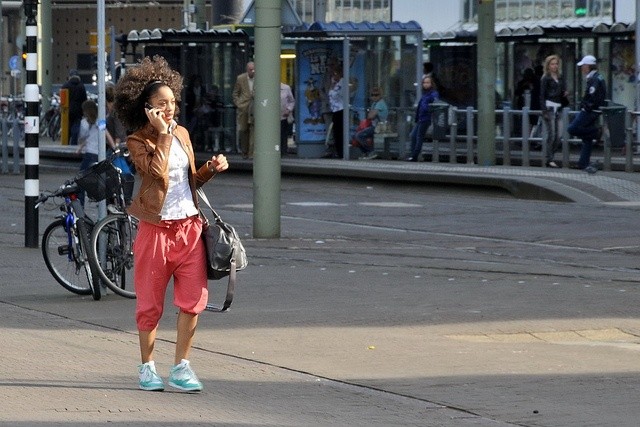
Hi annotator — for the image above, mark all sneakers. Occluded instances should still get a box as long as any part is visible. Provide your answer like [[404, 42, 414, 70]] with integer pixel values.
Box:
[[358, 150, 379, 160], [168, 358, 203, 392], [137, 360, 165, 390]]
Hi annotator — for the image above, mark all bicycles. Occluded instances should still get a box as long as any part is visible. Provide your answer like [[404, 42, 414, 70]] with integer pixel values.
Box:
[[79, 140, 140, 299], [0, 93, 62, 141], [31, 175, 101, 302]]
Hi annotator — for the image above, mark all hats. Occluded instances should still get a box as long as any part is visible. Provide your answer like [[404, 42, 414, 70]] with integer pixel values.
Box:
[[67, 71, 79, 80], [576, 55, 597, 66]]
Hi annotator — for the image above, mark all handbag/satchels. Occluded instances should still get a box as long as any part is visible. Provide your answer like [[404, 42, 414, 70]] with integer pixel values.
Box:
[[195, 186, 249, 312]]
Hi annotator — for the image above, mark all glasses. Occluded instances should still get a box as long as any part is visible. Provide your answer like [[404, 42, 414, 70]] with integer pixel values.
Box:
[[370, 93, 380, 97]]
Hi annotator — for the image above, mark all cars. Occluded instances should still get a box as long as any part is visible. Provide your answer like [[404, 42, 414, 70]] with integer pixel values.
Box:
[[53, 84, 96, 96]]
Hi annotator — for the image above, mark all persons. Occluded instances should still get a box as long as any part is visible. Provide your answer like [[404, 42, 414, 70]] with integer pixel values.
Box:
[[540, 54, 562, 168], [354, 86, 389, 160], [62, 70, 88, 146], [114, 54, 229, 394], [194, 85, 220, 151], [328, 68, 344, 158], [105, 87, 126, 156], [515, 68, 540, 136], [407, 74, 440, 162], [280, 82, 295, 158], [565, 55, 610, 171], [76, 100, 98, 209], [232, 62, 256, 154], [185, 73, 206, 152]]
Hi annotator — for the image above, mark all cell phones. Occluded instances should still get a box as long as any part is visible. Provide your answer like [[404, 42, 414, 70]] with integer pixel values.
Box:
[[145, 102, 160, 114]]
